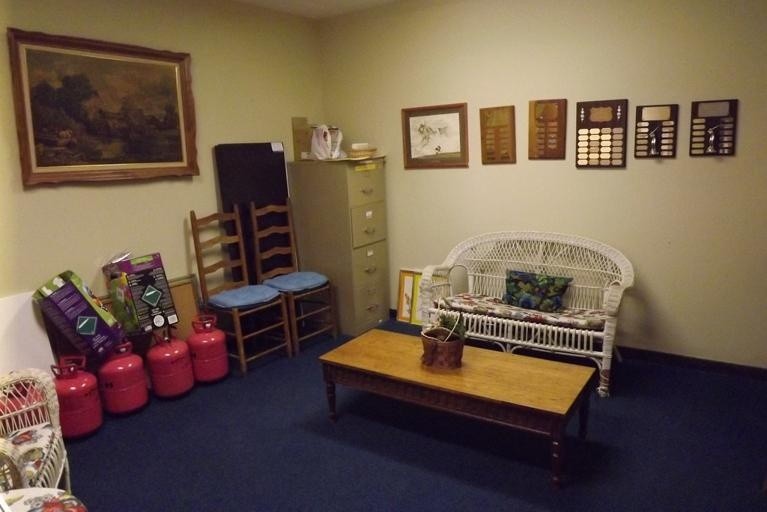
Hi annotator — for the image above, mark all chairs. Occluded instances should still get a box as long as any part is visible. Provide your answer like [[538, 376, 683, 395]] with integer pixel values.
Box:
[[0, 368, 71, 496], [191, 204, 292, 371], [251, 198, 337, 355], [0, 487, 86, 511]]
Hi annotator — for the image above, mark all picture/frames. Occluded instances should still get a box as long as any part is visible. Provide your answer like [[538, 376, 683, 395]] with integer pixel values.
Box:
[[396, 268, 415, 324], [409, 270, 447, 326], [400, 102, 469, 169], [6, 25, 199, 187]]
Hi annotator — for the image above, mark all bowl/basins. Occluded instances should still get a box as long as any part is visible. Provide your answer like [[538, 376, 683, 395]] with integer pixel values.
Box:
[[352, 143, 369, 150]]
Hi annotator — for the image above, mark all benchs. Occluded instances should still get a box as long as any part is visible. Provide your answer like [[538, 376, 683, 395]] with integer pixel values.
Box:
[[419, 231, 635, 397]]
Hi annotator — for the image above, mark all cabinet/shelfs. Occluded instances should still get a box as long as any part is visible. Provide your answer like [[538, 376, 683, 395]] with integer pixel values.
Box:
[[289, 158, 391, 336]]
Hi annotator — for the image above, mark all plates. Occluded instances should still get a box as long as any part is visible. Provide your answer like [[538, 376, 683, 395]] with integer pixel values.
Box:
[[349, 148, 376, 157]]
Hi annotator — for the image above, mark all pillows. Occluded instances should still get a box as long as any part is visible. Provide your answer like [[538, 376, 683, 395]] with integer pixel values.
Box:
[[502, 269, 574, 312]]
